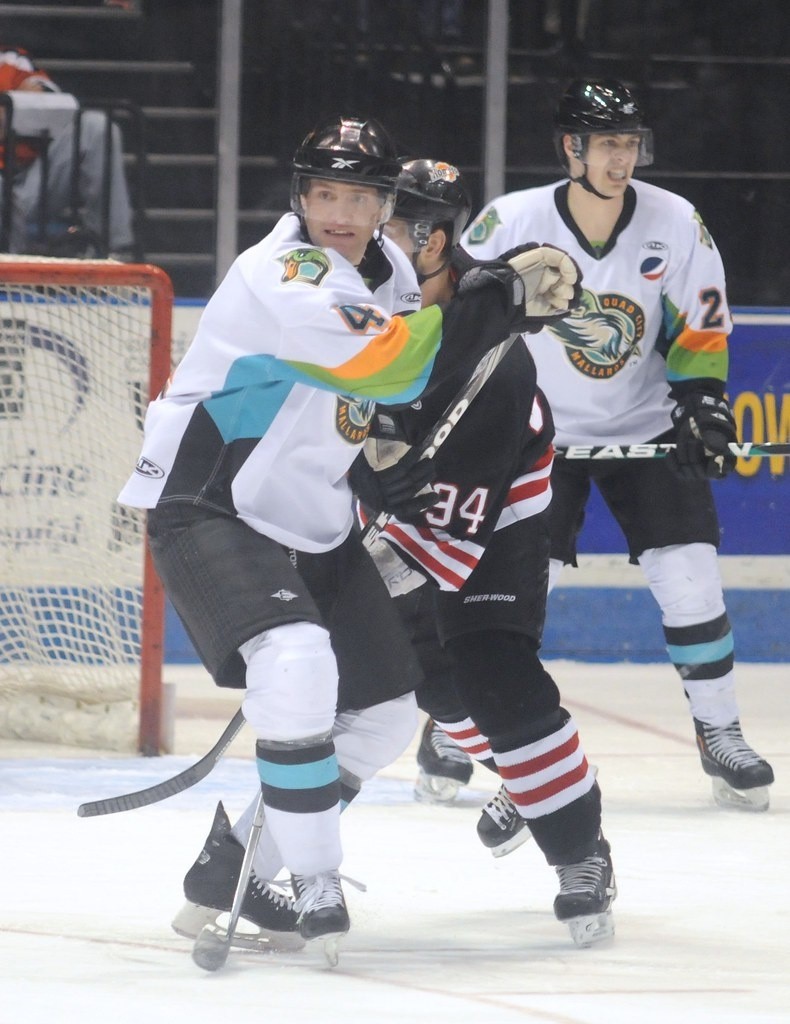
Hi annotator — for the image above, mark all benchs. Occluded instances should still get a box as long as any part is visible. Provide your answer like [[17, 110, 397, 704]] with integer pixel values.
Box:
[[0, 1, 790, 272]]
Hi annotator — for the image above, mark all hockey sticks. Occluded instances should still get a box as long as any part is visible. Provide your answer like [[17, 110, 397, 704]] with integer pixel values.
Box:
[[552, 443, 790, 459], [192, 788, 266, 973], [76, 707, 252, 820]]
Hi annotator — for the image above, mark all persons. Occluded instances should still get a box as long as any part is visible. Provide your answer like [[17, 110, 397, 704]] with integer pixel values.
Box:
[[347, 160, 619, 947], [117, 117, 583, 971], [0, 47, 135, 261], [415, 76, 774, 810]]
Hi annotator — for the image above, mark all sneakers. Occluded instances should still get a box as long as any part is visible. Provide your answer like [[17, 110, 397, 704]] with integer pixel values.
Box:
[[477, 763, 530, 858], [553, 831, 619, 942], [289, 868, 368, 969], [171, 801, 309, 956], [417, 714, 474, 802], [696, 719, 774, 811]]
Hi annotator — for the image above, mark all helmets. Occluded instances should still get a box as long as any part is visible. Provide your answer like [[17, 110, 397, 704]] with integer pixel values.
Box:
[[291, 112, 397, 225], [384, 158, 472, 250], [555, 82, 653, 168]]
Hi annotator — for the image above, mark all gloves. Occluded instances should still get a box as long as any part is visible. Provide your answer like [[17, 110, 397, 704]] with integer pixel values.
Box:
[[458, 242, 583, 332], [348, 437, 439, 520], [671, 376, 739, 476]]
